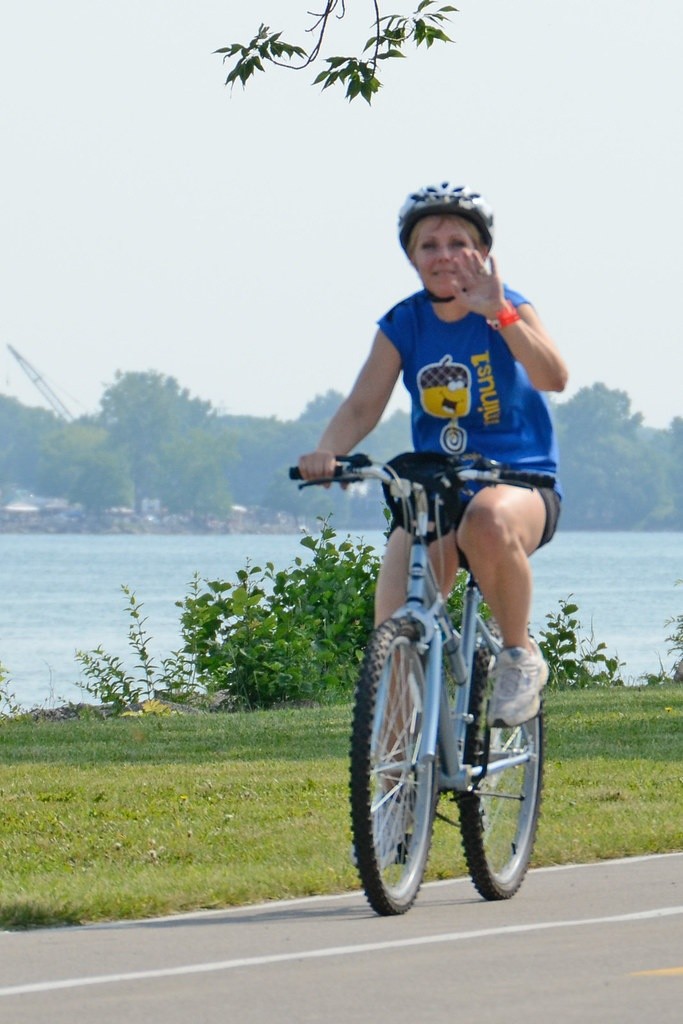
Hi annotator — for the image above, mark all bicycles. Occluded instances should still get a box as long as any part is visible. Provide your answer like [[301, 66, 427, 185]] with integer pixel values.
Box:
[[287, 451, 562, 919]]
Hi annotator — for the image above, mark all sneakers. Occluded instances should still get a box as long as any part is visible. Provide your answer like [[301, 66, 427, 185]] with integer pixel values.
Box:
[[352, 787, 417, 869], [486, 636, 550, 728]]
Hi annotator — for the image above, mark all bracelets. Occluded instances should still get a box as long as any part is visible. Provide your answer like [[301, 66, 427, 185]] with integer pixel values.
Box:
[[484, 298, 522, 331]]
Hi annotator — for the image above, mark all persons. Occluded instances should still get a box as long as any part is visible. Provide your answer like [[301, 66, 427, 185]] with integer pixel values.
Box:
[[293, 181, 570, 870]]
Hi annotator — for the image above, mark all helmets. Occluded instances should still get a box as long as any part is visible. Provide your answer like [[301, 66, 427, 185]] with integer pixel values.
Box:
[[398, 181, 494, 261]]
